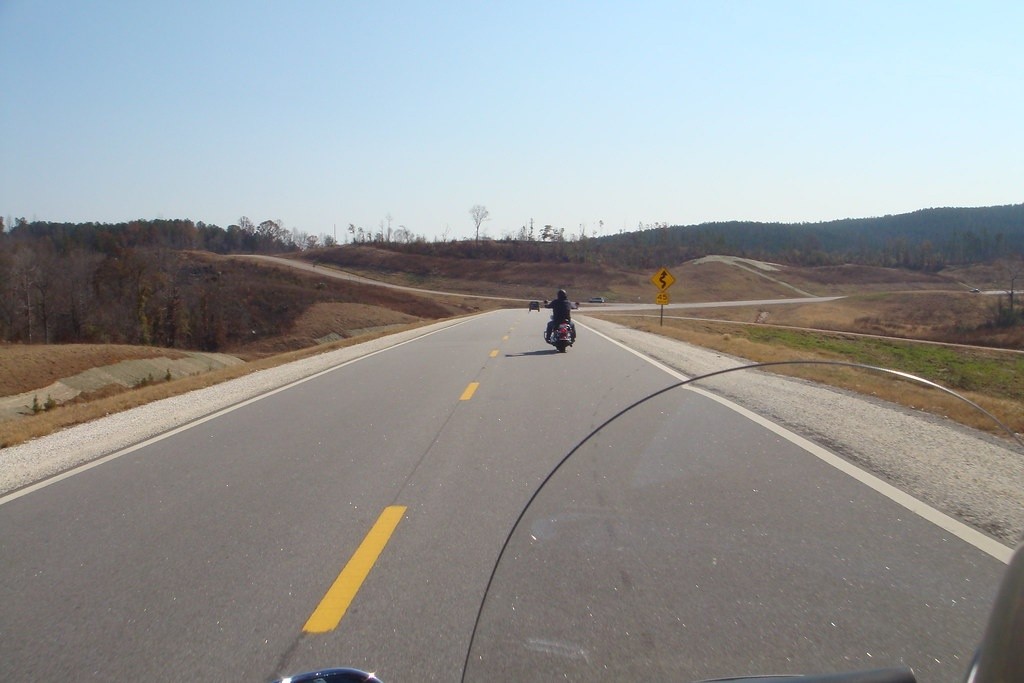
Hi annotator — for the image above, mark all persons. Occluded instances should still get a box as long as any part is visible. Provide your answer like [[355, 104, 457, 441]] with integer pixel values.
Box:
[[544, 289, 577, 341]]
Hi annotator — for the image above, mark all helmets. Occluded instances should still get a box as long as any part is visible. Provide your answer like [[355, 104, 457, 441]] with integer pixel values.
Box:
[[558, 290, 567, 299]]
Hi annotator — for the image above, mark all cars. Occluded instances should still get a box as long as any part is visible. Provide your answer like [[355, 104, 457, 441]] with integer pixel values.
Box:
[[589, 297, 604, 304], [529, 300, 541, 311]]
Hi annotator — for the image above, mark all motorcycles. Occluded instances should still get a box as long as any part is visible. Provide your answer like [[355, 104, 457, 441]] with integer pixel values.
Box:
[[541, 299, 580, 354]]
